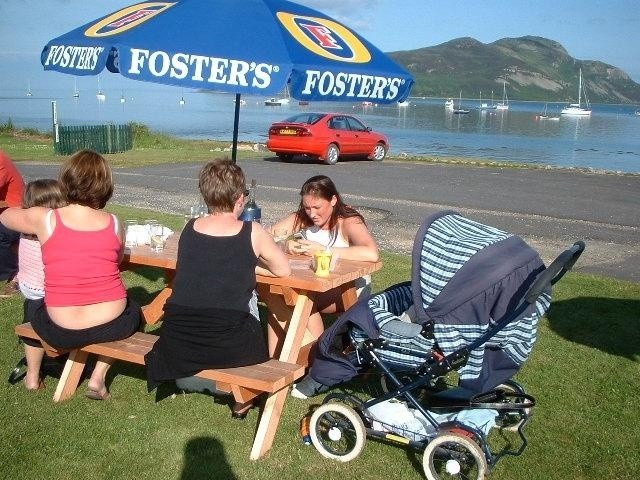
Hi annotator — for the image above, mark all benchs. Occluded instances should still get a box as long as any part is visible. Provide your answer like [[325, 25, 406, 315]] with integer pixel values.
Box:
[[13, 322, 306, 465]]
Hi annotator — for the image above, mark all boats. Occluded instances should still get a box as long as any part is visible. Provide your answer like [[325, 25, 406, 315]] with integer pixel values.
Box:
[[445, 98, 454, 108], [264, 98, 289, 106]]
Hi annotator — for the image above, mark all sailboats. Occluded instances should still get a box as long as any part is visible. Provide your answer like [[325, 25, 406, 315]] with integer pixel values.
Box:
[[540, 68, 591, 121], [476, 82, 509, 110], [455, 89, 471, 114]]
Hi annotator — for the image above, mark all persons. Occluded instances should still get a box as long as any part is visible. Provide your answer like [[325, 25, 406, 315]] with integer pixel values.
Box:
[[260, 174, 381, 363], [0, 148, 145, 403], [158, 157, 293, 421], [0, 146, 30, 285], [15, 178, 70, 324]]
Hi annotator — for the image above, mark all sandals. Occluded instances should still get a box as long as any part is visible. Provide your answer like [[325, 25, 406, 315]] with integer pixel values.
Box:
[[232, 398, 256, 420]]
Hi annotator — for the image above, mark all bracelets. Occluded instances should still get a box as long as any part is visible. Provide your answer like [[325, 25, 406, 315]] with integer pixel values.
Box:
[[325, 245, 330, 252]]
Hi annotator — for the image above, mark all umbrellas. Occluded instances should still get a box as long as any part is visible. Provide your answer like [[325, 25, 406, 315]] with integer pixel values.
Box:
[[39, 0, 419, 166]]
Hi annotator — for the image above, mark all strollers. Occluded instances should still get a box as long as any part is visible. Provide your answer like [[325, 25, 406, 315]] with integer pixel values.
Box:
[[308, 208, 583, 480]]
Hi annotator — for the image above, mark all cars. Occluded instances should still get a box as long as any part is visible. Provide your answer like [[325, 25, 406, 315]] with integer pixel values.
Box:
[[267, 113, 389, 164]]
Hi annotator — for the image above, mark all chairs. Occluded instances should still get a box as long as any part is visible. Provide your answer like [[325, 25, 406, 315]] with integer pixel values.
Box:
[[332, 120, 346, 129]]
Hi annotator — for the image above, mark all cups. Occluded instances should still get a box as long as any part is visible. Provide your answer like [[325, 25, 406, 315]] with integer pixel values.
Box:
[[145, 219, 163, 252], [123, 219, 138, 250], [311, 251, 332, 276], [274, 228, 286, 255]]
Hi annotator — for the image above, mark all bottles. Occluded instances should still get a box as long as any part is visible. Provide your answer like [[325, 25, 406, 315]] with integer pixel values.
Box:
[[240, 202, 261, 225], [301, 416, 312, 446]]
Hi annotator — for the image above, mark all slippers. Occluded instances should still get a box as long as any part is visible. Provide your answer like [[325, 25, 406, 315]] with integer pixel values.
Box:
[[85, 382, 112, 401], [37, 372, 46, 391]]
